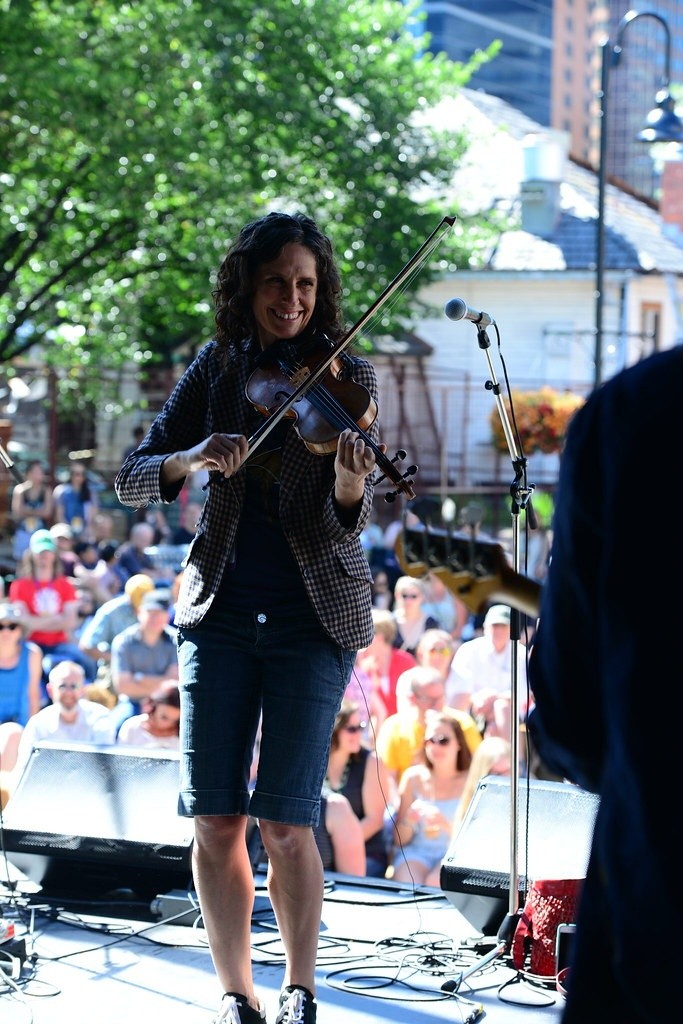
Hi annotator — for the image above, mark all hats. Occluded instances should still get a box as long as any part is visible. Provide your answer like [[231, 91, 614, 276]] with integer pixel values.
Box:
[[126, 575, 156, 605], [142, 590, 170, 610], [485, 604, 512, 625], [30, 529, 58, 553], [51, 522, 73, 541]]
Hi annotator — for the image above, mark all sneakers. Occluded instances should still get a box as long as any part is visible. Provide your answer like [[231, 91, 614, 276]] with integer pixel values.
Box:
[[272, 984, 317, 1024], [213, 991, 267, 1024]]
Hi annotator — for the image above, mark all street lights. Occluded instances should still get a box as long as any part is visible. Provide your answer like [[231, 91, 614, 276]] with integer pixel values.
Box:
[[587, 8, 683, 389]]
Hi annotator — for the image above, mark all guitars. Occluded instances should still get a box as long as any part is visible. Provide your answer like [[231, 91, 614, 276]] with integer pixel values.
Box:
[[390, 491, 546, 626]]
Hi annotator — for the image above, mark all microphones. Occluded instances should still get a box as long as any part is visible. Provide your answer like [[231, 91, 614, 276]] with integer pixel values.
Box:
[[444, 297, 496, 325]]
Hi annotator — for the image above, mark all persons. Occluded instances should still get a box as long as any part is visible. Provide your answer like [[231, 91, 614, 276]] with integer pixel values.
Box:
[[395, 628, 457, 713], [445, 605, 536, 777], [0, 521, 179, 811], [171, 502, 203, 546], [392, 714, 473, 888], [356, 609, 418, 718], [114, 210, 382, 1024], [534, 341, 683, 1024], [313, 701, 389, 878], [375, 666, 483, 857], [360, 496, 549, 614], [344, 666, 387, 749], [11, 458, 108, 579], [123, 426, 146, 464]]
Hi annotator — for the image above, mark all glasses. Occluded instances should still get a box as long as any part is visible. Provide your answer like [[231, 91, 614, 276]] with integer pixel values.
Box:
[[0, 622, 20, 630], [54, 681, 81, 690], [340, 720, 364, 733], [424, 735, 457, 745], [402, 592, 418, 601]]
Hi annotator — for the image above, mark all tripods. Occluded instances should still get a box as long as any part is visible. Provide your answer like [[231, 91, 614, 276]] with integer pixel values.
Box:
[[385, 311, 538, 992]]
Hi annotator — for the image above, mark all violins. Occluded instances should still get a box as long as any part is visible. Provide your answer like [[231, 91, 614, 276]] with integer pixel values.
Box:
[[243, 341, 422, 503]]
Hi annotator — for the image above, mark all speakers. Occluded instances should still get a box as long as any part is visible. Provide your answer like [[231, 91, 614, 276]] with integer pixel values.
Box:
[[0, 740, 262, 887], [439, 774, 601, 936]]
